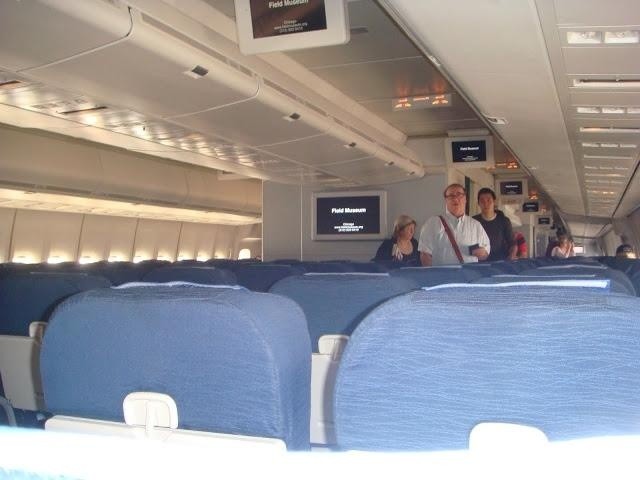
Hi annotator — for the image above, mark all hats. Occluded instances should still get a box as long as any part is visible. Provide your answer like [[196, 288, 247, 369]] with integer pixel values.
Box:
[[616, 244, 632, 252]]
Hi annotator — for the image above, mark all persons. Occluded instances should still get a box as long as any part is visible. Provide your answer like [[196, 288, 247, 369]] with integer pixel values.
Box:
[[504, 217, 528, 260], [417, 184, 490, 267], [550, 233, 577, 260], [373, 214, 423, 269], [471, 188, 515, 263]]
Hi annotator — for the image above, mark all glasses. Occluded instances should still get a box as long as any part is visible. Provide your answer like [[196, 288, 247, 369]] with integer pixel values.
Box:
[[445, 193, 465, 200]]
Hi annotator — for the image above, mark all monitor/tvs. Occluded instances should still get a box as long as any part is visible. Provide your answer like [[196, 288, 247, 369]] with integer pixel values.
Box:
[[231, 0, 350, 57], [310, 191, 386, 240], [522, 199, 542, 214], [536, 216, 558, 236], [494, 179, 528, 202], [444, 137, 495, 171]]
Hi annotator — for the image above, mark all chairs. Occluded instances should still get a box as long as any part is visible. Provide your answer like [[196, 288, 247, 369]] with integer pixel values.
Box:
[[0, 255, 640, 480]]
